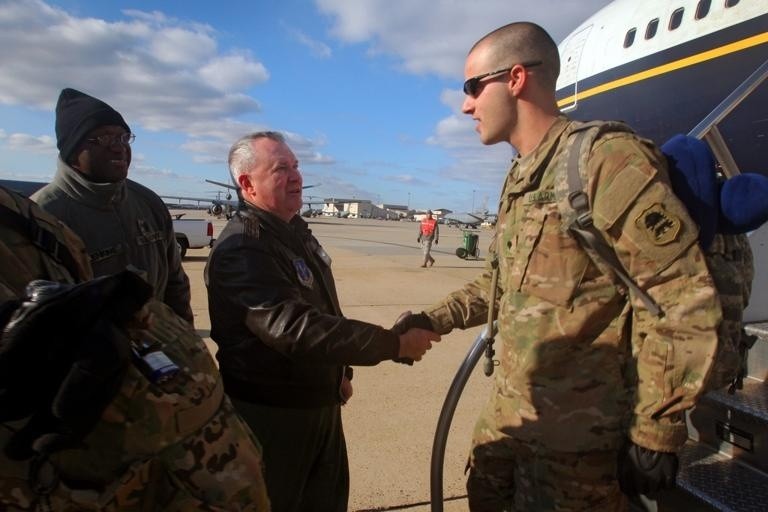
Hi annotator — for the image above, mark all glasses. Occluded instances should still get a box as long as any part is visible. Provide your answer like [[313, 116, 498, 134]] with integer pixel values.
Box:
[[463, 61, 541, 96], [87, 134, 135, 146]]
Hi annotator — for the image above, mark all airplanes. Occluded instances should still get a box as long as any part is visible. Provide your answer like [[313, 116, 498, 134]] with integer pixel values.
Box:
[[547, 1, 768, 339], [445, 210, 498, 231], [158, 179, 372, 219]]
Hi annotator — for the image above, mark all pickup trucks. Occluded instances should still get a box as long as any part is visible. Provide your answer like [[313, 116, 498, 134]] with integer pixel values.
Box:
[[170, 218, 215, 260]]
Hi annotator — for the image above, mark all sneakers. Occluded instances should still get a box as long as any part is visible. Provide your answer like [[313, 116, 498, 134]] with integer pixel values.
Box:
[[421, 260, 435, 267]]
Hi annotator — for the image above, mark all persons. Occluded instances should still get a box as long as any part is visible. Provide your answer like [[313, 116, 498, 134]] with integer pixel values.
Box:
[[388, 21, 756, 509], [202, 127, 442, 511], [27, 85, 195, 336], [0, 184, 274, 510], [416, 208, 440, 268]]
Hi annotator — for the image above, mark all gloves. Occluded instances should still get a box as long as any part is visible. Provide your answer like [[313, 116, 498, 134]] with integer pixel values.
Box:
[[617, 442, 678, 500], [392, 312, 434, 365], [417, 237, 420, 242], [435, 239, 438, 244]]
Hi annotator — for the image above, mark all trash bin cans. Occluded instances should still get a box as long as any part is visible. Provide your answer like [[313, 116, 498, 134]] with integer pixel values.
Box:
[[464, 234, 478, 260]]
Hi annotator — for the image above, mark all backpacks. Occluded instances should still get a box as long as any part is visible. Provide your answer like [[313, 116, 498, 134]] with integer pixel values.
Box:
[[570, 120, 754, 394]]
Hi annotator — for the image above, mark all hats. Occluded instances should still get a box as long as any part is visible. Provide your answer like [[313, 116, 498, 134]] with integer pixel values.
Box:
[[55, 88, 131, 163], [426, 210, 433, 214]]
